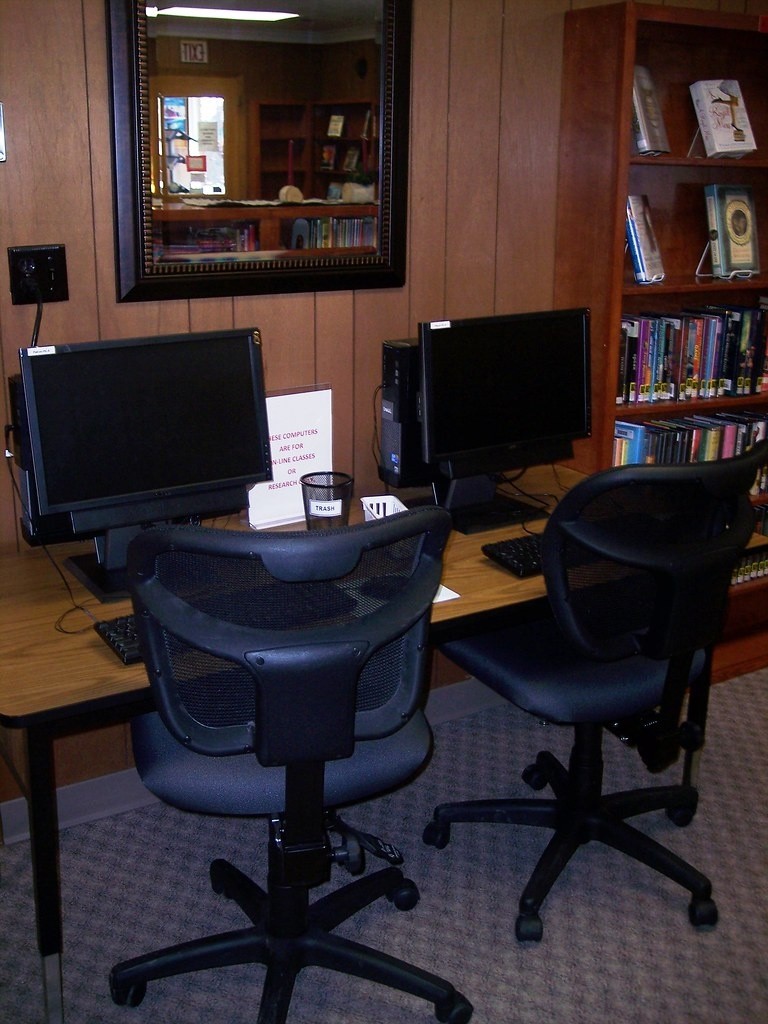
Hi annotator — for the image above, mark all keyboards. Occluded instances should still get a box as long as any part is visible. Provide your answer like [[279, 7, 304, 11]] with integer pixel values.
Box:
[[479, 533, 548, 578], [95, 579, 358, 664]]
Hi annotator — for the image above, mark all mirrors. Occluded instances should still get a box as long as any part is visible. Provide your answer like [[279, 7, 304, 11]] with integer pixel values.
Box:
[[104, 0, 416, 304]]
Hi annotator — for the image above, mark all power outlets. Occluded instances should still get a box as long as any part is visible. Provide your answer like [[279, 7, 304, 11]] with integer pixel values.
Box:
[[7, 244, 70, 305]]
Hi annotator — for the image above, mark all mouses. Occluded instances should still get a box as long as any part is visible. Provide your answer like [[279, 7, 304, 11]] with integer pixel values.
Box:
[[362, 571, 409, 599]]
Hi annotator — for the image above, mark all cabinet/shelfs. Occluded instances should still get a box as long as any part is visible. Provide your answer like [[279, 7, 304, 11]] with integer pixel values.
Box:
[[248, 97, 379, 201], [151, 203, 378, 261], [552, 0, 768, 505]]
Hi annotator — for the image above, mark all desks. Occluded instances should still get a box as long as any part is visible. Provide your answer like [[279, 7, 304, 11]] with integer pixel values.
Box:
[[0, 464, 768, 1024]]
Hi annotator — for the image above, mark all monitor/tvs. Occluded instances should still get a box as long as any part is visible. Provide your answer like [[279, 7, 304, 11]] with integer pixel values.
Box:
[[414, 307, 591, 535], [16, 325, 273, 606]]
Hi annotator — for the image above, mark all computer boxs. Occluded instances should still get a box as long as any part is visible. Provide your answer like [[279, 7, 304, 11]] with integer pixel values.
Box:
[[9, 368, 257, 547], [379, 337, 432, 490]]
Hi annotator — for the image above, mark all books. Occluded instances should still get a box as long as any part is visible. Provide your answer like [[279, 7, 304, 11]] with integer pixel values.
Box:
[[613, 411, 768, 586], [614, 296, 768, 404], [321, 145, 335, 169], [702, 180, 760, 276], [628, 65, 670, 154], [343, 146, 361, 171], [311, 217, 376, 248], [156, 220, 254, 254], [328, 110, 376, 140], [621, 195, 663, 283], [689, 80, 757, 158]]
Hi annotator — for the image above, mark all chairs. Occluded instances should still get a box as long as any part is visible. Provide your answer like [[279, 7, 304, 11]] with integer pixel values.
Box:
[[421, 439, 768, 942], [109, 503, 475, 1024]]
